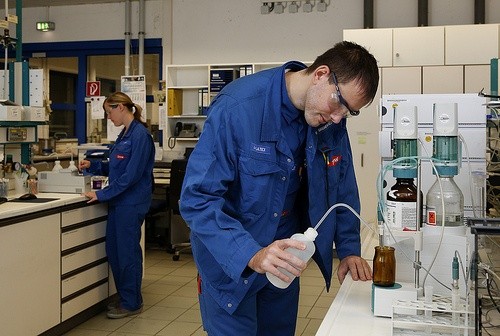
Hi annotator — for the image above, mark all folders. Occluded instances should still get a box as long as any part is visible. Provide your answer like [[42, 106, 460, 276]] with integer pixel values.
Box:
[[198, 89, 208, 116]]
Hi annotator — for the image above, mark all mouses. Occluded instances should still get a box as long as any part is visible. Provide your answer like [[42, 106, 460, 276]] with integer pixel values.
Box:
[[18, 193, 37, 200]]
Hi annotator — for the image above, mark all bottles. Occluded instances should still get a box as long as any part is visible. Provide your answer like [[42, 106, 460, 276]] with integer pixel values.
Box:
[[68, 161, 78, 173], [52, 160, 63, 173], [266, 228, 318, 289], [386, 168, 423, 229], [426, 165, 464, 227], [93, 176, 102, 191]]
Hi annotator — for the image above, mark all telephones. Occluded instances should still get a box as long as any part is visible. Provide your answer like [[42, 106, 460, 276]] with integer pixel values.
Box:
[[174, 122, 197, 138]]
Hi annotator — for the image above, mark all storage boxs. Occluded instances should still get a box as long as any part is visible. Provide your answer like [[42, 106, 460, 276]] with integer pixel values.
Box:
[[54, 138, 79, 155], [371, 282, 416, 318]]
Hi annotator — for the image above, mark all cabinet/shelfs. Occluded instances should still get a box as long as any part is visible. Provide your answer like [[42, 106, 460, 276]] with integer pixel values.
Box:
[[0, 193, 145, 336], [165, 60, 314, 140], [342, 24, 500, 248], [145, 162, 172, 244], [0, 0, 50, 165]]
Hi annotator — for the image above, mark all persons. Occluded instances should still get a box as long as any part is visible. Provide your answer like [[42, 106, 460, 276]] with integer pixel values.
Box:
[[79, 92, 156, 318], [179, 41, 379, 336]]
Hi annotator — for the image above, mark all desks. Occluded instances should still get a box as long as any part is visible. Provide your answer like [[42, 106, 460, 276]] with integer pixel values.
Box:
[[315, 223, 415, 335]]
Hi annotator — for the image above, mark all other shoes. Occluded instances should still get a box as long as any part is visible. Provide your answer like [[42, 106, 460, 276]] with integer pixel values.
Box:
[[107, 300, 143, 309], [106, 306, 141, 318]]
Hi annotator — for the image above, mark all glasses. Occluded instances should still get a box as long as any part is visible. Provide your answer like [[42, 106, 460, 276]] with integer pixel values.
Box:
[[327, 71, 359, 118], [105, 106, 116, 116]]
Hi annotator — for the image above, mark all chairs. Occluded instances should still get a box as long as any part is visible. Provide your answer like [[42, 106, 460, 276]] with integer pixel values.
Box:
[[166, 159, 192, 262]]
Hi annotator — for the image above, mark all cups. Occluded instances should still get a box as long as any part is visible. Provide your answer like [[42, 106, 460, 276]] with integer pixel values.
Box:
[[373, 246, 395, 287]]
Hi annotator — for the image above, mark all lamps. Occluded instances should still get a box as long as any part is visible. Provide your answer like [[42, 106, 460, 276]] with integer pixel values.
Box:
[[275, 0, 288, 14], [260, 0, 275, 15], [317, 0, 331, 12], [303, 0, 316, 13], [288, 0, 302, 14]]
